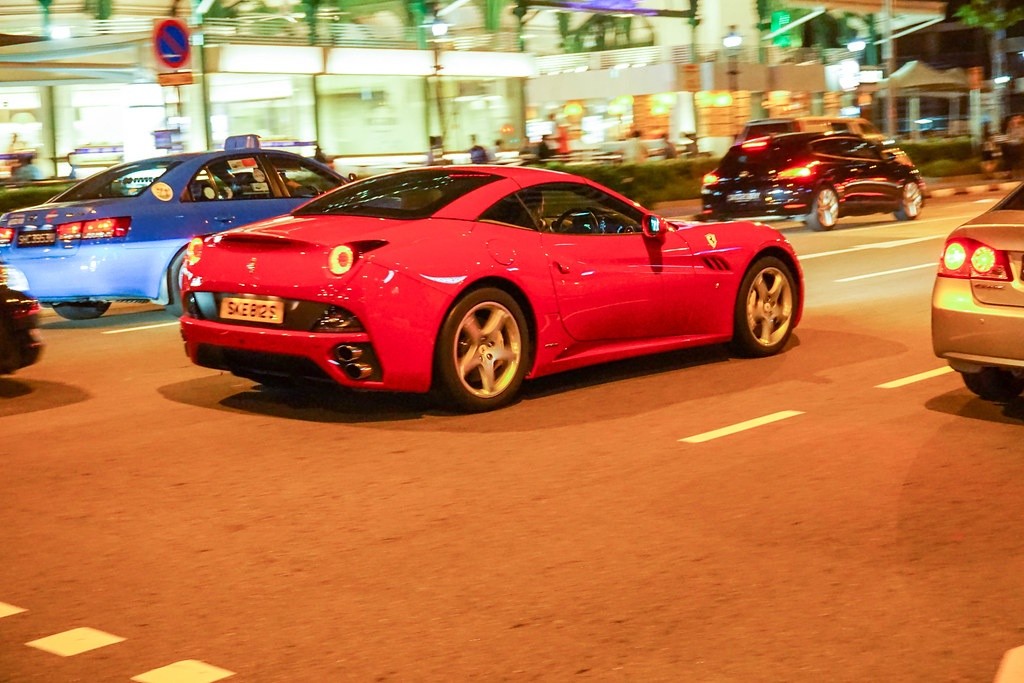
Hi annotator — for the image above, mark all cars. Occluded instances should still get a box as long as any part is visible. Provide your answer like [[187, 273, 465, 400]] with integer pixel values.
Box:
[[182, 163, 806, 412], [931, 183, 1024, 404], [1, 133, 357, 320], [699, 129, 926, 232]]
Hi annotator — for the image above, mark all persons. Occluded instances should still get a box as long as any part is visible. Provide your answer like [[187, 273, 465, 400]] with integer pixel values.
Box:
[[538, 134, 549, 159], [470, 134, 487, 164], [1002, 113, 1023, 179], [623, 130, 647, 163], [12, 154, 40, 180], [980, 114, 996, 181], [660, 132, 676, 160], [524, 190, 545, 228]]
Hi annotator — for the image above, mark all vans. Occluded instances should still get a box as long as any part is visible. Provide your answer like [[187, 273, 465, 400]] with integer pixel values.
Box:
[[733, 115, 888, 145]]
